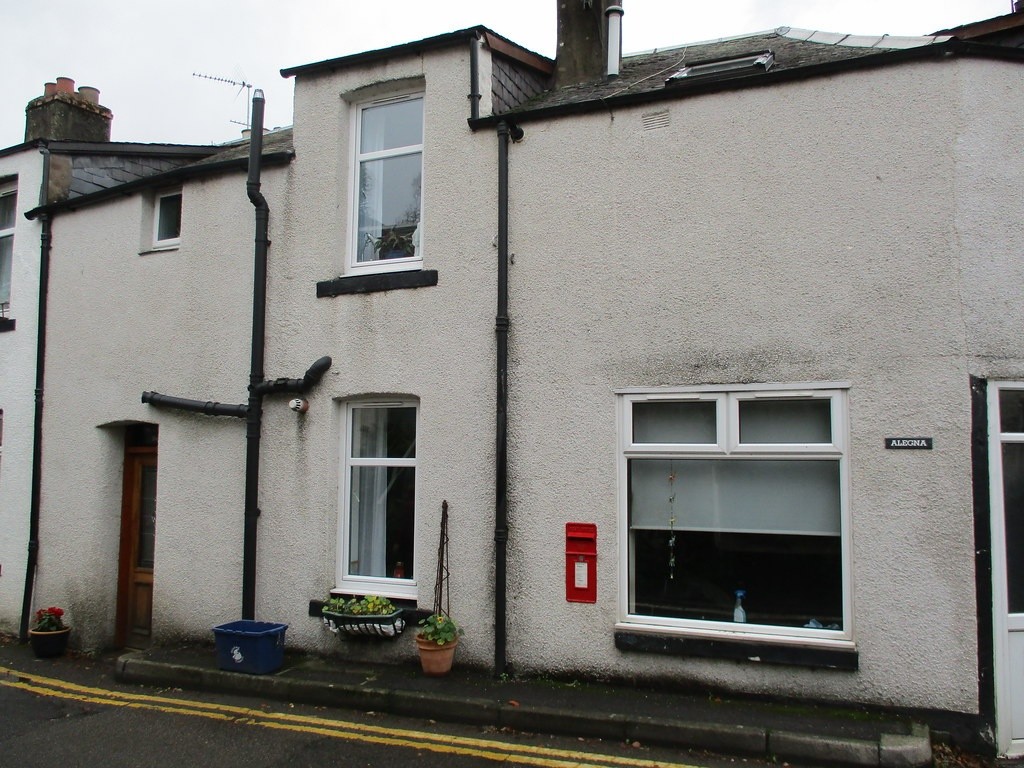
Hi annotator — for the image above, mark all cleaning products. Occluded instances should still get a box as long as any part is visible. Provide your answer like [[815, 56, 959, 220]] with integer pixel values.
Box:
[[733, 590, 747, 623]]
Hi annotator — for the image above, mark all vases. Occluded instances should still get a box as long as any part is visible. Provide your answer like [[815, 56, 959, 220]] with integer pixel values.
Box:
[[323, 596, 400, 625], [415, 634, 458, 674], [28, 627, 72, 659]]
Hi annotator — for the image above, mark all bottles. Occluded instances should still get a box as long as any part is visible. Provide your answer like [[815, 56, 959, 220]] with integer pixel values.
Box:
[[394, 562, 404, 578], [734, 591, 749, 623]]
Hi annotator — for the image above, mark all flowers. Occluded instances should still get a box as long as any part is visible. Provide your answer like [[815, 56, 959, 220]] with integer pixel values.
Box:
[[33, 607, 64, 632], [419, 614, 465, 644]]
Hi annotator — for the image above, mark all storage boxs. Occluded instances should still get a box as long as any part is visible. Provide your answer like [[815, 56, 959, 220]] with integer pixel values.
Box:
[[211, 619, 288, 675]]
